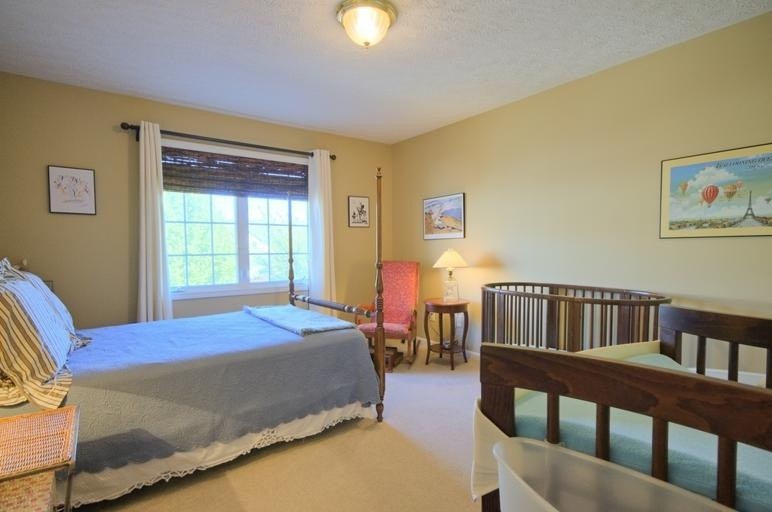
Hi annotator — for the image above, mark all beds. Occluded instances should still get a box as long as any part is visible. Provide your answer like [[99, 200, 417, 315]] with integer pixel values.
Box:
[[0, 167, 387, 510], [478, 304, 772, 511]]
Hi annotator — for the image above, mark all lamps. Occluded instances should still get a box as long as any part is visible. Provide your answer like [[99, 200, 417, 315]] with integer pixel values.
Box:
[[431, 248, 471, 303], [334, 0, 399, 50]]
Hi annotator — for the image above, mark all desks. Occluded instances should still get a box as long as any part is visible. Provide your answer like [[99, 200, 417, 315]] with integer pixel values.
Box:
[[0, 404, 82, 511], [423, 297, 469, 370]]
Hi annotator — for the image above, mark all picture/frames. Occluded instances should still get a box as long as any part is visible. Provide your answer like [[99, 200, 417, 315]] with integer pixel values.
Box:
[[657, 142, 772, 240], [422, 192, 465, 239], [347, 195, 370, 228], [47, 164, 98, 217]]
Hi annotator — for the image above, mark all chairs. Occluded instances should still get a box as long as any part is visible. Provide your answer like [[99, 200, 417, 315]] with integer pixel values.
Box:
[[354, 259, 421, 366]]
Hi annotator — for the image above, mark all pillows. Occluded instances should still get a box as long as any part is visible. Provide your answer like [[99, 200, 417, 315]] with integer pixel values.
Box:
[[0, 253, 95, 412]]
